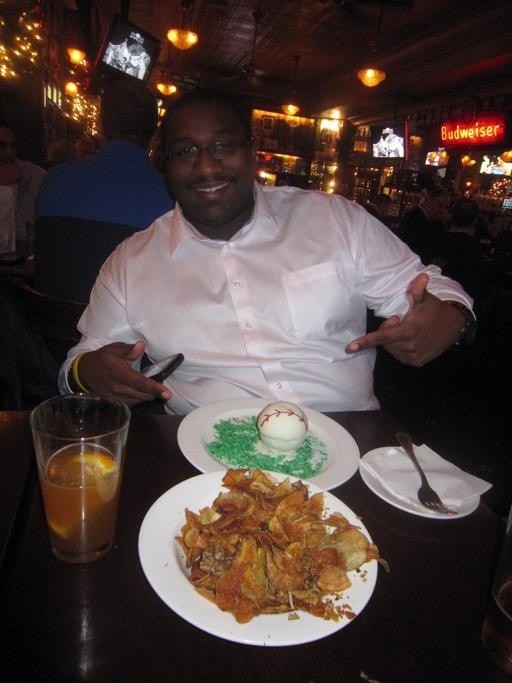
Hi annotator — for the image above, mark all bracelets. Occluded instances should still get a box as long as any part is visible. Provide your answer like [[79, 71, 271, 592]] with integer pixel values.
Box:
[[72, 352, 91, 393]]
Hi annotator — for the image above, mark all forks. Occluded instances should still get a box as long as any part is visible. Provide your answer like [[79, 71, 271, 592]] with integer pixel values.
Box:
[[395, 431, 460, 516]]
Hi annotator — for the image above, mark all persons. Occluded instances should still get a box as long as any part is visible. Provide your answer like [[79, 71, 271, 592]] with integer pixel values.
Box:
[[362, 193, 393, 222], [1, 119, 49, 245], [416, 171, 451, 222], [423, 200, 486, 305], [57, 88, 478, 416], [37, 83, 174, 231]]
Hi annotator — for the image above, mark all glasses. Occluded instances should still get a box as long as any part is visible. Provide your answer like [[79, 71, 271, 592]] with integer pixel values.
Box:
[[176, 134, 242, 167]]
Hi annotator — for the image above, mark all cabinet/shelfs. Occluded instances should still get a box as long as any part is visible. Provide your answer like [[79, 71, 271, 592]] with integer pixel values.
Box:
[[349, 121, 512, 234], [251, 108, 345, 195]]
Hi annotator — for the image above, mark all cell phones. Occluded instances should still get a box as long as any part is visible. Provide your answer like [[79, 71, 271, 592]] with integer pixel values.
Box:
[[142, 353, 184, 383]]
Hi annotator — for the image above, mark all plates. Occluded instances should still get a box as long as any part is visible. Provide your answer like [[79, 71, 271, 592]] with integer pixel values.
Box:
[[176, 397, 361, 492], [359, 445, 481, 520], [138, 468, 379, 647]]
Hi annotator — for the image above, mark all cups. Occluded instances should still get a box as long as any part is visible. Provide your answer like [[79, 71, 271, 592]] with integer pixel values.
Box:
[[31, 394, 130, 564]]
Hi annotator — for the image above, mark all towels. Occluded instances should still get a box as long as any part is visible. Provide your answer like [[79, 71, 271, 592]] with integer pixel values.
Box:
[[362, 440, 492, 514]]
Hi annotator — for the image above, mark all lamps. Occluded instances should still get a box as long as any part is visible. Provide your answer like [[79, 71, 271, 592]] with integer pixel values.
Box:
[[157, 81, 176, 95], [238, 63, 267, 78], [281, 92, 302, 116], [167, 24, 198, 50], [68, 47, 86, 62], [357, 55, 386, 87]]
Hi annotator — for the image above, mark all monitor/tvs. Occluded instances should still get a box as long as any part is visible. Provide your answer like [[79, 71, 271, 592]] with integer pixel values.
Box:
[[370, 120, 408, 161], [93, 13, 162, 87]]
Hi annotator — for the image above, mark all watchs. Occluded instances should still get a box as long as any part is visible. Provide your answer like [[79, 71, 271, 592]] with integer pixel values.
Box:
[[442, 301, 478, 349]]
